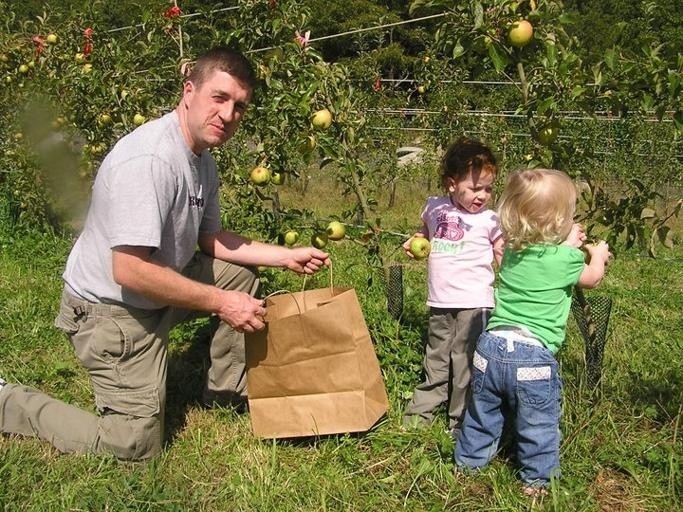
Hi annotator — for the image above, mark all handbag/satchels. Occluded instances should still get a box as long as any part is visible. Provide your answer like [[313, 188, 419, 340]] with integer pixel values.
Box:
[[244, 260, 390, 441]]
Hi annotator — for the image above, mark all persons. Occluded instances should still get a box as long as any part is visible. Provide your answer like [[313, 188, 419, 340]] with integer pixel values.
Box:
[[401, 141, 506, 444], [0, 46, 333, 464], [448, 168, 616, 502]]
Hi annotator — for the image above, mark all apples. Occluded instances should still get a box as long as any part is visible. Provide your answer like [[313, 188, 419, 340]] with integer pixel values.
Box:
[[537, 128, 555, 146], [418, 85, 426, 94], [508, 20, 533, 47], [409, 237, 431, 259], [0, 34, 347, 250]]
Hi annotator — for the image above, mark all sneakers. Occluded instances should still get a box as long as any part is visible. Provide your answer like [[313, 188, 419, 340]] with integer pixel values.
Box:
[[203, 388, 247, 414]]
